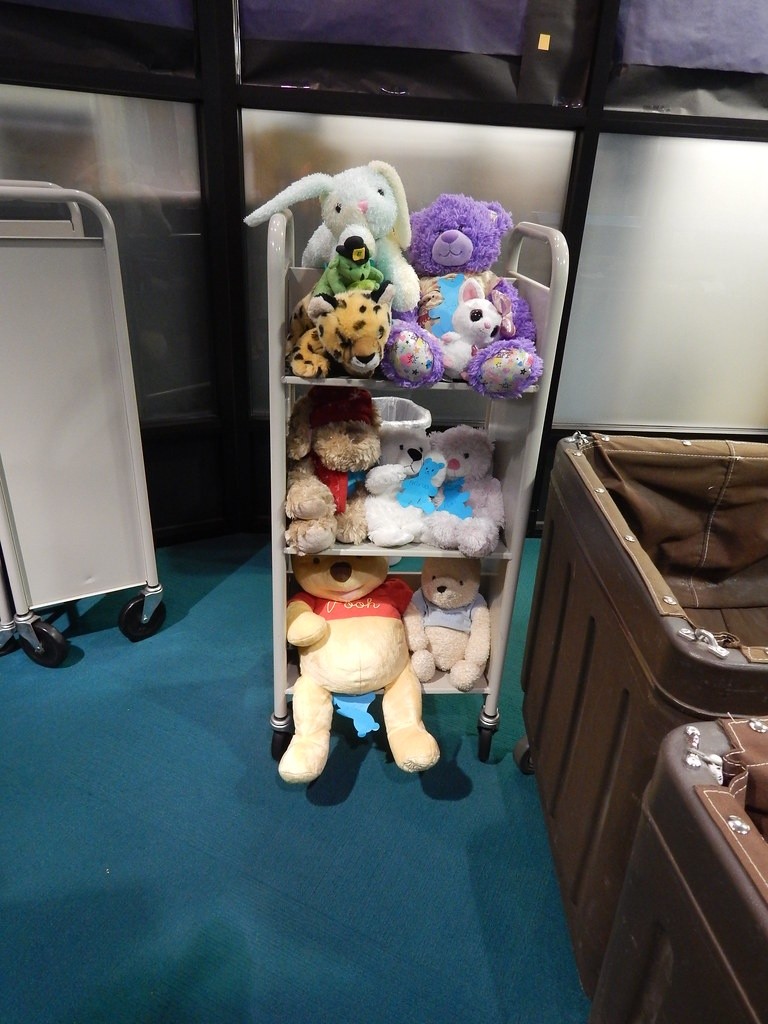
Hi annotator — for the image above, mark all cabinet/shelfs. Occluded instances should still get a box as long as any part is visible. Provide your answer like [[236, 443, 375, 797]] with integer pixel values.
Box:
[[269, 206, 568, 761], [1, 180, 166, 661]]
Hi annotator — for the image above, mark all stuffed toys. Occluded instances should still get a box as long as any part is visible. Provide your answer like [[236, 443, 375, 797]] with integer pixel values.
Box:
[[244, 160, 542, 782]]
